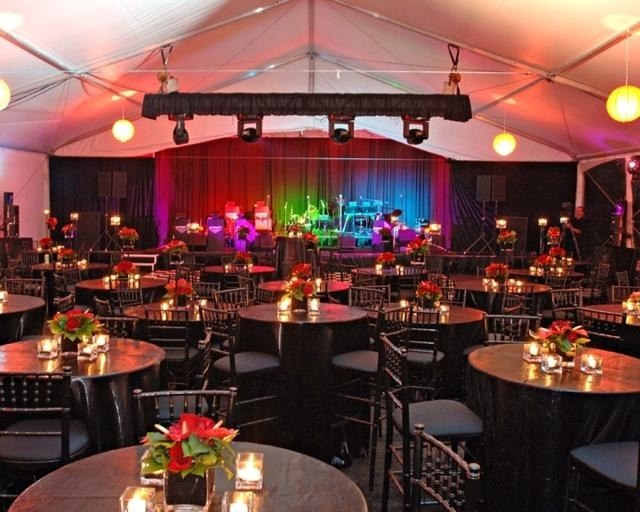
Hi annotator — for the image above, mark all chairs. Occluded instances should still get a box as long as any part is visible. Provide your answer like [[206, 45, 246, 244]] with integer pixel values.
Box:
[[324, 326, 411, 440], [199, 305, 289, 441], [379, 330, 484, 512], [564, 438, 638, 510], [1, 365, 93, 512], [408, 423, 481, 508], [2, 209, 640, 398]]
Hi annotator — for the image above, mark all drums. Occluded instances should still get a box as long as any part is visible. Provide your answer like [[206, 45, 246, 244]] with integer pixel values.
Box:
[[355, 218, 367, 233], [370, 212, 376, 226]]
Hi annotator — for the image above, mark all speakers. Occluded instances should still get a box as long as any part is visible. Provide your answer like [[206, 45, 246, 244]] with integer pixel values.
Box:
[[112, 171, 128, 199], [73, 212, 101, 256], [504, 216, 527, 254], [489, 174, 507, 203], [337, 235, 356, 252], [178, 234, 207, 250], [250, 235, 273, 252], [96, 171, 111, 198], [475, 173, 491, 202]]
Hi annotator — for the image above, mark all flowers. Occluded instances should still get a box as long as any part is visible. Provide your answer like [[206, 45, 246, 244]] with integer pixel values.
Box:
[[136, 411, 239, 481]]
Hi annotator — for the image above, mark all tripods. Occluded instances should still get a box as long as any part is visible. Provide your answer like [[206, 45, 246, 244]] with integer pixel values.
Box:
[[89, 198, 123, 253], [104, 199, 124, 253], [557, 224, 583, 262], [463, 203, 494, 254], [477, 202, 498, 257]]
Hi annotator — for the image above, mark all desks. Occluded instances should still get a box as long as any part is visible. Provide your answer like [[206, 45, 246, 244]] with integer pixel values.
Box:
[[8, 444, 367, 511]]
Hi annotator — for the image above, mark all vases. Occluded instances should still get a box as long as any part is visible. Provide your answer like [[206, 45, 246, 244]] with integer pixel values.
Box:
[[163, 465, 216, 509]]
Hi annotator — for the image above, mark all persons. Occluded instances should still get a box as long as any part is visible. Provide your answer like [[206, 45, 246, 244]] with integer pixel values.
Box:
[[385, 210, 401, 225], [570, 205, 591, 261]]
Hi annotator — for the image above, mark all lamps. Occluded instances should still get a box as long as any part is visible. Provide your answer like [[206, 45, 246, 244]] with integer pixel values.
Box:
[[606, 25, 639, 122], [112, 97, 135, 143], [492, 99, 517, 158], [141, 92, 474, 151]]
